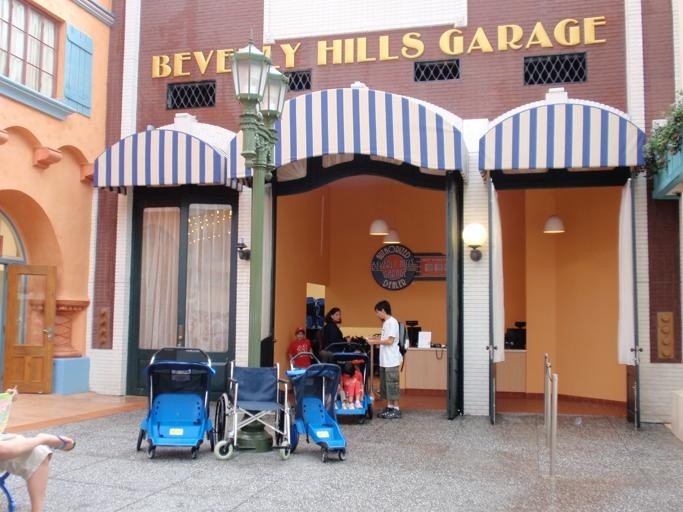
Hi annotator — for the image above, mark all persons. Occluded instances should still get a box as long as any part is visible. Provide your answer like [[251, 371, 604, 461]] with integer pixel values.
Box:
[[333, 385, 346, 411], [338, 361, 364, 411], [287, 326, 314, 408], [322, 307, 351, 363], [364, 299, 402, 418], [318, 324, 329, 362], [0, 387, 75, 512]]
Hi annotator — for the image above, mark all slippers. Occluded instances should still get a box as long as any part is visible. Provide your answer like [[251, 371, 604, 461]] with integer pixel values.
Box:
[[36, 433, 76, 451]]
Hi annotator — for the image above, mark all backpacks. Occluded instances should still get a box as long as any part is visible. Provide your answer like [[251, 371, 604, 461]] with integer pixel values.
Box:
[[398, 320, 410, 355]]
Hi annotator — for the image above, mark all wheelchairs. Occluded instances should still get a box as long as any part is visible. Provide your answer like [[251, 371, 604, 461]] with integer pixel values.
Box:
[[214, 360, 292, 460]]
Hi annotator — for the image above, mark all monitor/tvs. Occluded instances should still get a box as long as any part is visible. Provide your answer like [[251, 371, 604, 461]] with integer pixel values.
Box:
[[506, 328, 526, 349]]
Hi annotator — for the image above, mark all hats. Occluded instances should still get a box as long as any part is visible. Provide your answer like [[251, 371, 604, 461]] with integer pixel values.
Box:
[[295, 327, 305, 335]]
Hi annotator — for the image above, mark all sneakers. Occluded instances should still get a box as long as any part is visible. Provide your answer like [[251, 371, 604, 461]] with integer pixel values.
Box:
[[377, 407, 401, 419]]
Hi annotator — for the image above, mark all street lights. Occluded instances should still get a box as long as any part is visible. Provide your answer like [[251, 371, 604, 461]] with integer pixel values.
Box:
[[230, 31, 290, 454]]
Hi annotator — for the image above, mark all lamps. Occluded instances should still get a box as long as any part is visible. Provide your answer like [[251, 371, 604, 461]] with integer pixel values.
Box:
[[461, 221, 490, 262], [234, 237, 251, 261], [368, 164, 400, 246], [541, 174, 567, 236]]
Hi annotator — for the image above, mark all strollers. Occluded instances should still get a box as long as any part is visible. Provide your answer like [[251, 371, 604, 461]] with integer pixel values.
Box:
[[291, 352, 346, 462], [137, 347, 215, 459], [319, 342, 373, 424]]
